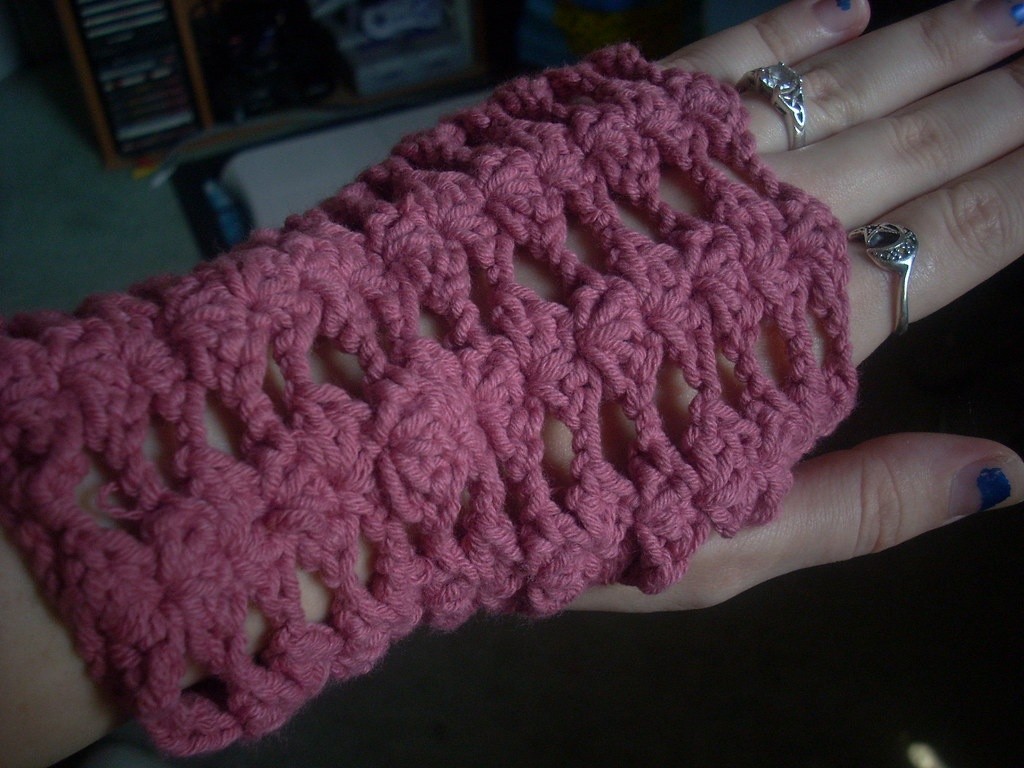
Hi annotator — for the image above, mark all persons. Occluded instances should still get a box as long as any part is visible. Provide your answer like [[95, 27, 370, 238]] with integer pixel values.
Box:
[[0, 0, 1024, 768]]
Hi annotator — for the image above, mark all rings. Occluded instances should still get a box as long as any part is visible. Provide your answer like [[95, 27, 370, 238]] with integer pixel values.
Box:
[[848, 222, 918, 335], [735, 61, 806, 149]]
